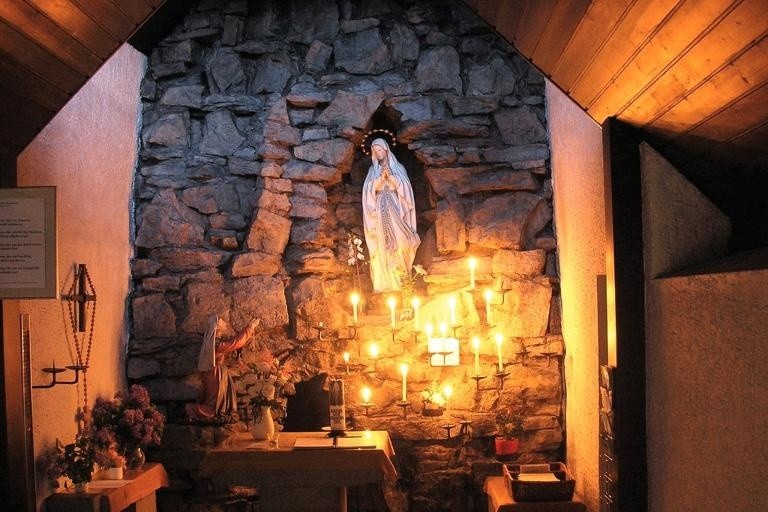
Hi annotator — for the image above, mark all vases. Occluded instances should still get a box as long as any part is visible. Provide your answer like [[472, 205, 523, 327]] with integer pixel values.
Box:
[[74, 482, 89, 494], [250, 405, 275, 441], [495, 437, 520, 455]]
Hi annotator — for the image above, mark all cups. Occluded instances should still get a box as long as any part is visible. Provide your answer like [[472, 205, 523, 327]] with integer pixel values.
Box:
[[264, 430, 281, 448]]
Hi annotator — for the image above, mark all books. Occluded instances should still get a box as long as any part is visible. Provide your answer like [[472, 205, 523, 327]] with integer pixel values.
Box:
[[292, 437, 377, 449], [598, 364, 615, 435]]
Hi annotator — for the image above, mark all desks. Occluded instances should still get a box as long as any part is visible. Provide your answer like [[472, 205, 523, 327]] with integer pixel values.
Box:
[[486, 476, 586, 511], [46, 462, 161, 512], [211, 432, 392, 512]]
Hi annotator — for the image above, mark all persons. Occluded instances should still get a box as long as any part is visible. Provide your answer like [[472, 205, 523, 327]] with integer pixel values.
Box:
[[186, 310, 260, 422], [362, 137, 423, 296]]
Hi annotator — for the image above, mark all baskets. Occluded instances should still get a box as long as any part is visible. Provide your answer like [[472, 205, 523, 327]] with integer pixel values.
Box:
[[503, 462, 577, 502]]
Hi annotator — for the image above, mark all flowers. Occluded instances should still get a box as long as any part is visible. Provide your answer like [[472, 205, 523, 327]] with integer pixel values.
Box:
[[495, 412, 533, 440], [38, 384, 167, 488], [231, 358, 296, 425], [347, 231, 365, 266]]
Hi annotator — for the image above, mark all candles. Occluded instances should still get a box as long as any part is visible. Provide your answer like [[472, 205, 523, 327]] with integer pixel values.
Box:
[[334, 258, 519, 407]]
